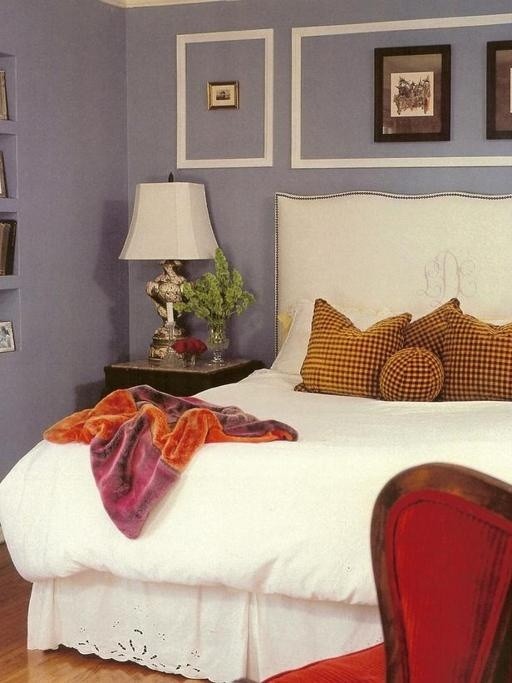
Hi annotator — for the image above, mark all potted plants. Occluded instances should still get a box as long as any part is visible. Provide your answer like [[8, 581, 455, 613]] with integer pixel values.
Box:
[[207, 81, 239, 110], [0, 71, 10, 120], [0, 321, 16, 353], [374, 44, 451, 142], [0, 150, 8, 198], [486, 40, 512, 139]]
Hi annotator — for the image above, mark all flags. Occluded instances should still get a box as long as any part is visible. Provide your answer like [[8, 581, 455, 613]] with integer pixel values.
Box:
[[180, 352, 197, 366]]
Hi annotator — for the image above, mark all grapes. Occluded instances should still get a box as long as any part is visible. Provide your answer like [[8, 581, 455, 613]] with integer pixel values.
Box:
[[104, 358, 265, 397]]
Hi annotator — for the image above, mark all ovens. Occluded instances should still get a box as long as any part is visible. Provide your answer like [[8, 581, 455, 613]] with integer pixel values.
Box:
[[1, 190, 512, 683]]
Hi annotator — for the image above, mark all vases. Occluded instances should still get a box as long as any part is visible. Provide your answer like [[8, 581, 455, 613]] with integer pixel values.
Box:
[[170, 335, 207, 365]]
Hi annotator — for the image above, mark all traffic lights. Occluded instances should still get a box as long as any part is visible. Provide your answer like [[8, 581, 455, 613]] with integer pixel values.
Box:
[[380, 348, 444, 401], [443, 310, 512, 401], [293, 298, 412, 398], [407, 298, 463, 360]]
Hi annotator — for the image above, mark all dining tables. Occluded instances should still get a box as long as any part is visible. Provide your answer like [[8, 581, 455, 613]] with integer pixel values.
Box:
[[174, 247, 255, 366]]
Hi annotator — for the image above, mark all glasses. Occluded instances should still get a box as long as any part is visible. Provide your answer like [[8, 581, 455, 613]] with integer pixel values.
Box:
[[118, 172, 223, 365]]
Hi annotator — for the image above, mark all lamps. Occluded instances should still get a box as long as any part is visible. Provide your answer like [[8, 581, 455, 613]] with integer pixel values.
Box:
[[261, 462, 511, 683]]
